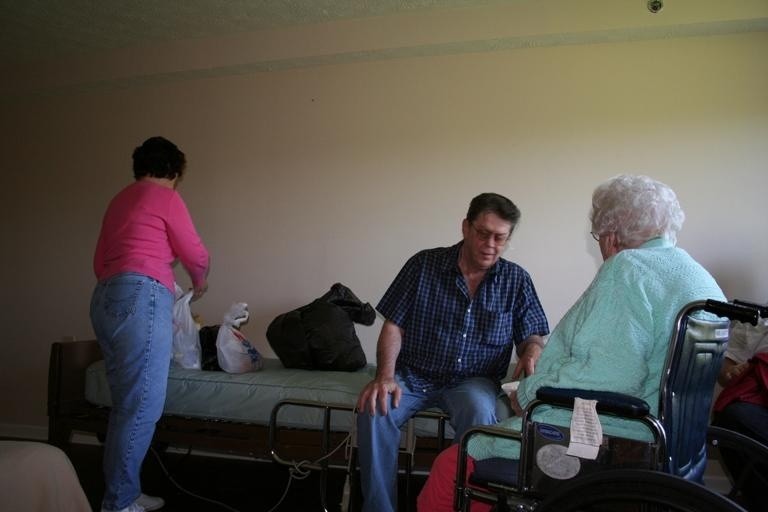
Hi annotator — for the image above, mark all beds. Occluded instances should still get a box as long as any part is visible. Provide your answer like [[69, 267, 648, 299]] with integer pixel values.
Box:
[[47, 339, 527, 512]]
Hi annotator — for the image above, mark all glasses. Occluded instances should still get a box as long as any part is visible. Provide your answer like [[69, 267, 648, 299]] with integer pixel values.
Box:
[[471, 222, 512, 246]]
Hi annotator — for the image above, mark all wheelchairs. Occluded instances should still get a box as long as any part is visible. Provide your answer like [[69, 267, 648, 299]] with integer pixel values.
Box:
[[453, 297, 767, 512]]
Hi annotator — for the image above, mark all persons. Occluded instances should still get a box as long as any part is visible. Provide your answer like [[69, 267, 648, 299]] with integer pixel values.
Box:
[[355, 193, 550, 511], [87, 135, 211, 512], [413, 173, 731, 511]]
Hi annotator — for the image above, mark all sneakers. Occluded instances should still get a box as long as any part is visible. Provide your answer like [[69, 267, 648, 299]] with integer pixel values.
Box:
[[100, 490, 166, 512]]
